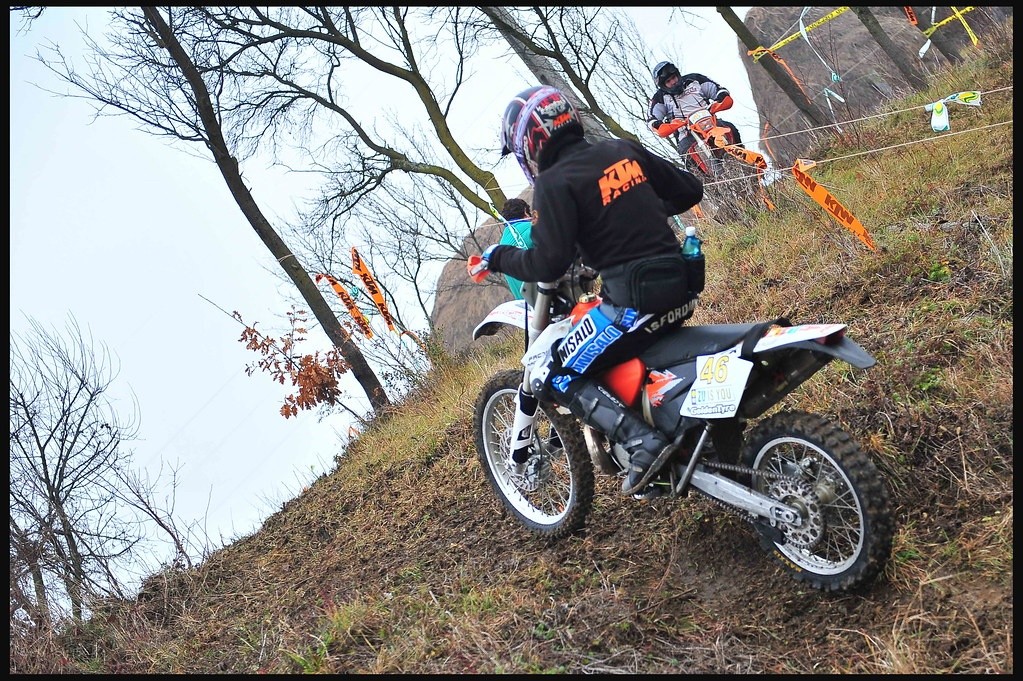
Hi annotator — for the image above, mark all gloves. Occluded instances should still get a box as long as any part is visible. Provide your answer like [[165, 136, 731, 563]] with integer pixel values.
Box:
[[481, 243, 500, 271], [716, 92, 729, 103], [652, 120, 663, 129]]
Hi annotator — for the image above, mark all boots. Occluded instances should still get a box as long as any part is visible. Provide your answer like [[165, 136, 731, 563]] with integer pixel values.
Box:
[[568, 379, 674, 496]]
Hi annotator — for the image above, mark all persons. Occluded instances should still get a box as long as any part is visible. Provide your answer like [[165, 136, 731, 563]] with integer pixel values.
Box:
[[479, 84, 707, 500], [497, 197, 586, 303], [646, 61, 744, 173]]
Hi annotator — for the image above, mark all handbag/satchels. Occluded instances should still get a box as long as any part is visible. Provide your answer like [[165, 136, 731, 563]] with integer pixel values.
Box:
[[625, 252, 705, 320]]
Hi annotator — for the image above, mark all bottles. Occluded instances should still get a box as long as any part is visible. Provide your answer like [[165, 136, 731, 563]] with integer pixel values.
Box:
[[681, 226, 701, 258]]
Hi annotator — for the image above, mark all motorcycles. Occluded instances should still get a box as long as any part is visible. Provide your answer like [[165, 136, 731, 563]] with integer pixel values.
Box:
[[467, 255, 892, 595], [657, 97, 758, 208]]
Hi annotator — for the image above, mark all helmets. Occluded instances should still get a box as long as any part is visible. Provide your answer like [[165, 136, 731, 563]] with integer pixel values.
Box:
[[653, 61, 684, 95], [501, 86, 584, 187]]
